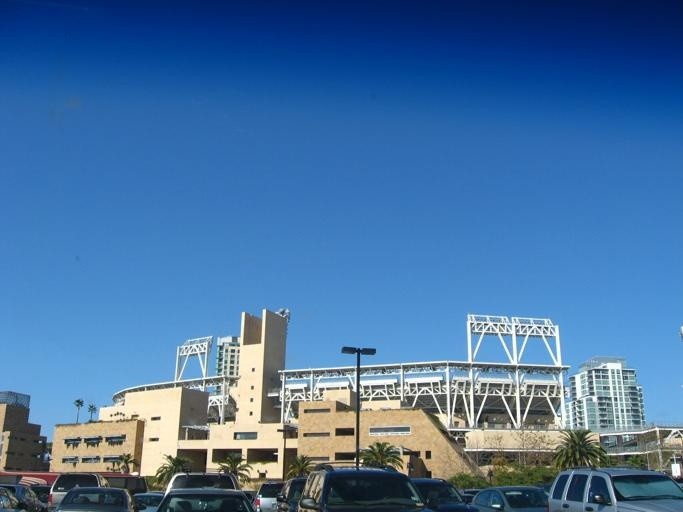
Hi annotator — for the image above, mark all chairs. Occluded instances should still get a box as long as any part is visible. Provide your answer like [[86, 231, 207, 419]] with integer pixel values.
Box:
[[72, 496, 119, 505], [327, 481, 355, 503], [173, 499, 238, 512]]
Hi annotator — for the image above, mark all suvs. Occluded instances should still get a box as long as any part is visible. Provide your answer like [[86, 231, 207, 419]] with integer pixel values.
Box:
[[298, 462, 431, 512], [548, 466, 683, 512]]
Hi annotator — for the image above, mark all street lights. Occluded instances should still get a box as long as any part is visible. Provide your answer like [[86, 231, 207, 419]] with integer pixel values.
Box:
[[277, 427, 293, 482], [340, 345, 377, 469]]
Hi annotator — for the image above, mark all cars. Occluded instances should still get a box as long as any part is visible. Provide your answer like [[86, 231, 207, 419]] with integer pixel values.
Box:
[[252, 481, 280, 512], [276, 477, 309, 512], [410, 477, 473, 512], [0, 471, 256, 512], [459, 488, 481, 504], [467, 485, 549, 512]]
